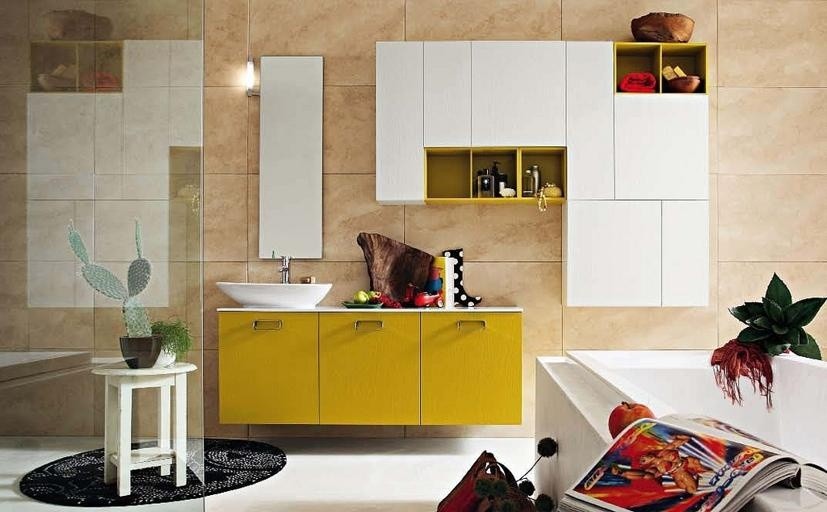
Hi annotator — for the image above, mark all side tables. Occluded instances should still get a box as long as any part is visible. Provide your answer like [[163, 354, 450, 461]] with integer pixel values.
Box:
[[90, 362, 199, 498]]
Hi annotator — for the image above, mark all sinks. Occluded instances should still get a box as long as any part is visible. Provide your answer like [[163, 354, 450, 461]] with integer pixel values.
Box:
[[216, 282, 333, 310]]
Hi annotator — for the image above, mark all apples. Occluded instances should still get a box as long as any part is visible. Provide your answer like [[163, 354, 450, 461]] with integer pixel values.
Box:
[[368, 291, 381, 304], [353, 290, 370, 304], [608, 401, 656, 440]]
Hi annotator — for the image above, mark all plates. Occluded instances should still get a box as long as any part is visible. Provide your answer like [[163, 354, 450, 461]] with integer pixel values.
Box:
[[341, 300, 383, 309]]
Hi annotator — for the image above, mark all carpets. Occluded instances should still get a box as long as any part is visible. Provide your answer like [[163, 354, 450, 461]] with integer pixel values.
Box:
[[19, 438, 287, 507]]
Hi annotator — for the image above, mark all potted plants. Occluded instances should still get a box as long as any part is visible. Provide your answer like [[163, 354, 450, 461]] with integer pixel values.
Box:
[[65, 215, 162, 369], [151, 317, 192, 368]]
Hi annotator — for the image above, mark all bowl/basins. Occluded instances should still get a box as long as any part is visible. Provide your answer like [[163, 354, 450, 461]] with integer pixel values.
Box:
[[500, 187, 517, 198], [669, 76, 701, 92]]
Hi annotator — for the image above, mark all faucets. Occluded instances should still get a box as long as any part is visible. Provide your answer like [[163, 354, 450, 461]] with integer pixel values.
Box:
[[278, 256, 293, 283]]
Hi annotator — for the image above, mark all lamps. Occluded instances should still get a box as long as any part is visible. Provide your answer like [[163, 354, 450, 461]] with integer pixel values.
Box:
[[244, 53, 260, 98]]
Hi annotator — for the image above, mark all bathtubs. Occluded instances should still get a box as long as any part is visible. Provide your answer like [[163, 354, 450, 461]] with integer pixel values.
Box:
[[534, 347, 827, 511]]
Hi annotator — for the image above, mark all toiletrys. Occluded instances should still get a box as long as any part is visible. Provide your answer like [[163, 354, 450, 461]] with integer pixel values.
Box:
[[475, 159, 509, 199]]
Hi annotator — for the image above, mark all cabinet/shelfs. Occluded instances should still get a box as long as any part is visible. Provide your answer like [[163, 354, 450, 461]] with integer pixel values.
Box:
[[375, 40, 711, 308], [216, 307, 319, 425], [420, 308, 524, 427], [318, 307, 420, 426]]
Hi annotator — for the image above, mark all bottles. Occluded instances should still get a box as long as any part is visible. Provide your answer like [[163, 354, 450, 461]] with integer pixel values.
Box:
[[474, 160, 507, 198], [524, 162, 542, 199]]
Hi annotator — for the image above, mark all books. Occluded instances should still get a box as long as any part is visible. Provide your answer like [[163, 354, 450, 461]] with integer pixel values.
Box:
[[558, 414, 826, 512]]
